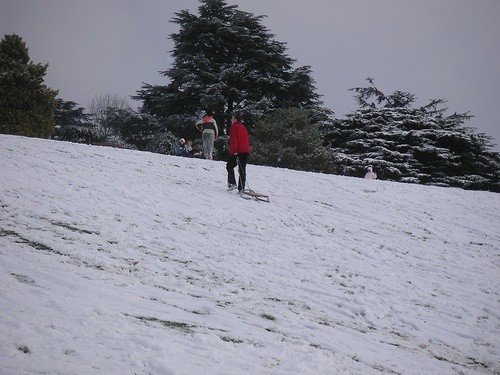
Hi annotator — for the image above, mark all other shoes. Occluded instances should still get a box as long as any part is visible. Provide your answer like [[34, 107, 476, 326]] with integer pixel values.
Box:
[[204, 157, 213, 161], [227, 183, 246, 195]]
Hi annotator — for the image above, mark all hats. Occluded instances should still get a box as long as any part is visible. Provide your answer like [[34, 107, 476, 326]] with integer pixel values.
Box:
[[178, 138, 185, 142]]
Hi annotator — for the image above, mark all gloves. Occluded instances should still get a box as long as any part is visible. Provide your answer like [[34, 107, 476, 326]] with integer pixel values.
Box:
[[233, 154, 240, 165]]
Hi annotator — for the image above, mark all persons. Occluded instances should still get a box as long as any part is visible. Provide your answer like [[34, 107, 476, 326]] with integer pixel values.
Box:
[[195, 113, 218, 160], [176, 138, 199, 156], [226, 114, 249, 192], [365, 167, 376, 180]]
